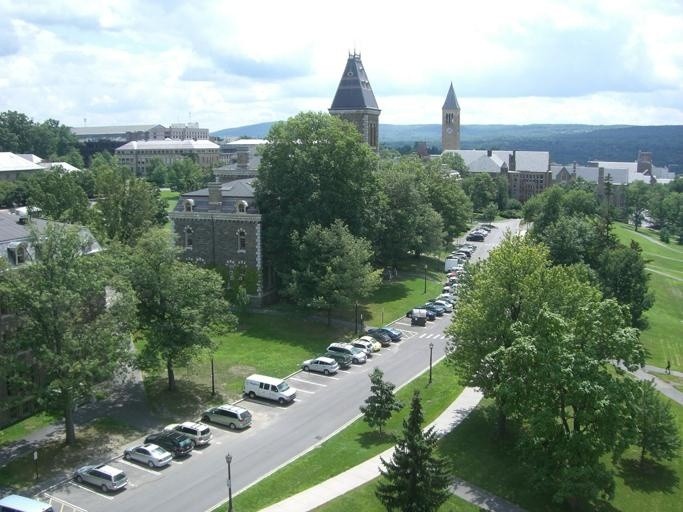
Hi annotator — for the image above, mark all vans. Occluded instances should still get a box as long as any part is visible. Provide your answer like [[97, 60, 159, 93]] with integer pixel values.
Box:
[[245, 373, 297, 404], [1, 495, 54, 512]]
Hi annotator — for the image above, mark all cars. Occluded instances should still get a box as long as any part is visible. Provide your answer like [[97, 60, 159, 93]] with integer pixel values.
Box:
[[408, 271, 464, 325], [466, 222, 494, 241], [445, 243, 477, 273], [203, 404, 252, 430], [144, 429, 194, 459], [324, 326, 402, 368], [302, 357, 338, 374], [124, 443, 172, 467]]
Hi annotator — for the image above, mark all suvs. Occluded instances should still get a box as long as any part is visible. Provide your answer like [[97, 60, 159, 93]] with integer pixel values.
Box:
[[74, 464, 128, 492], [164, 421, 213, 446]]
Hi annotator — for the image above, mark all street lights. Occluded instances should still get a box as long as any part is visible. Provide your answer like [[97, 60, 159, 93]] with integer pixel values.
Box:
[[424, 264, 427, 293], [210, 351, 214, 395], [225, 453, 232, 511], [430, 342, 433, 383]]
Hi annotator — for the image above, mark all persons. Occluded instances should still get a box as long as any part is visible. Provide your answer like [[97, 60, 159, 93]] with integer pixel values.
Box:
[[665, 361, 671, 374]]
